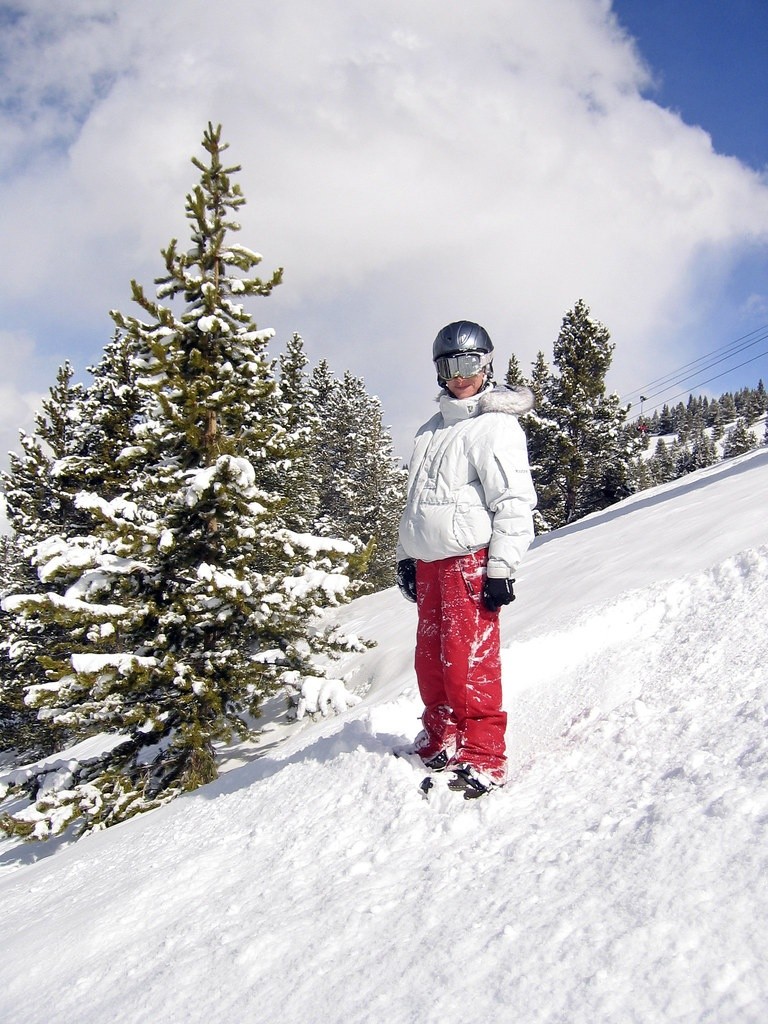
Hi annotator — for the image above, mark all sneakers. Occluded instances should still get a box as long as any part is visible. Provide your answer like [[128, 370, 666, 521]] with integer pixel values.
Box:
[[418, 757, 507, 800], [391, 737, 449, 772]]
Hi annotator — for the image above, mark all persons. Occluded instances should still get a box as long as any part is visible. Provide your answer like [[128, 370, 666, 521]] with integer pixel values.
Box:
[[394, 321, 538, 799]]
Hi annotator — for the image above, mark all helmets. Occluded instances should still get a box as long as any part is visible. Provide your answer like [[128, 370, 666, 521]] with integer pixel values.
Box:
[[432, 321, 493, 361]]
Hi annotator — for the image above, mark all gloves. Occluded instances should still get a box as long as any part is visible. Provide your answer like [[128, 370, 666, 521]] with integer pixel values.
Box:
[[397, 558, 416, 603], [483, 578, 516, 612]]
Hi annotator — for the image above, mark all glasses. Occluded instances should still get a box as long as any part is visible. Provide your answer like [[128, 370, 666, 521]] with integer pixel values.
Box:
[[435, 350, 494, 380]]
